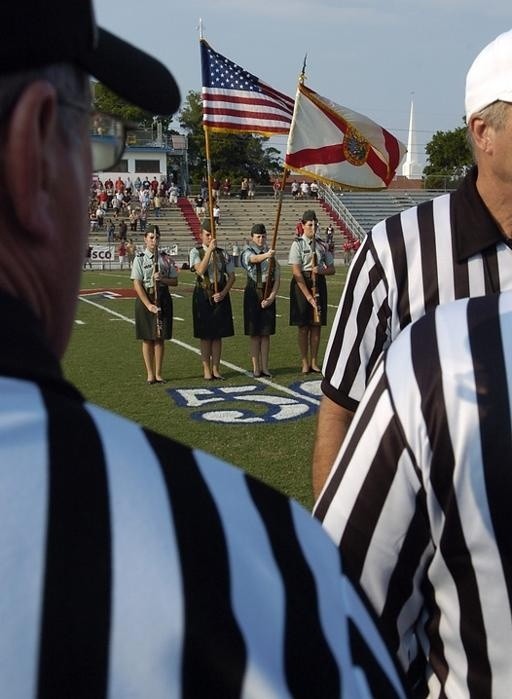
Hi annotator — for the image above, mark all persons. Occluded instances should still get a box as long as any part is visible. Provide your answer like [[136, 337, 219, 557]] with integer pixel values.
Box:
[[317, 222, 322, 239], [248, 178, 256, 200], [240, 178, 248, 199], [190, 218, 236, 381], [299, 179, 309, 200], [201, 177, 209, 199], [343, 240, 351, 266], [311, 26, 512, 508], [288, 209, 336, 376], [311, 289, 512, 699], [310, 180, 318, 200], [127, 239, 136, 270], [232, 241, 239, 267], [291, 180, 300, 199], [241, 224, 281, 379], [242, 240, 250, 251], [87, 176, 180, 244], [83, 244, 94, 272], [213, 204, 221, 226], [212, 178, 220, 199], [117, 239, 125, 271], [224, 179, 232, 199], [206, 193, 216, 215], [273, 179, 282, 200], [130, 225, 179, 384], [352, 237, 360, 257], [194, 193, 207, 219], [0, 0, 411, 699], [325, 223, 334, 244]]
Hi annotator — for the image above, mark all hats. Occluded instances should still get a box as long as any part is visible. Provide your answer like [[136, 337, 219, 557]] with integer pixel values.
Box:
[[251, 224, 266, 234], [303, 209, 316, 220], [202, 219, 216, 232], [465, 28, 512, 123], [0, 0, 180, 117], [146, 225, 160, 236]]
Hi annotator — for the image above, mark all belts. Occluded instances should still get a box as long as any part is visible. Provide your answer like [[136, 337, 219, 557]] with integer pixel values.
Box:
[[247, 282, 274, 289], [196, 282, 226, 290], [145, 287, 169, 294], [302, 271, 312, 278]]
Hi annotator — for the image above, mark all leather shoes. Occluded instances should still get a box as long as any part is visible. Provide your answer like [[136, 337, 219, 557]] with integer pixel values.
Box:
[[301, 368, 310, 375], [253, 371, 262, 378], [146, 376, 155, 385], [214, 373, 226, 381], [203, 374, 213, 381], [156, 379, 167, 385], [262, 371, 275, 379], [311, 366, 322, 374]]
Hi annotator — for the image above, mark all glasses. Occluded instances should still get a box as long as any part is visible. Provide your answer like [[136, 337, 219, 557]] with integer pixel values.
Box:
[[53, 96, 128, 174]]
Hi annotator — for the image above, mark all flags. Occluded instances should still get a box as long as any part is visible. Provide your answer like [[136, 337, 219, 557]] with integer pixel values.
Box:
[[283, 78, 408, 193], [199, 38, 295, 136]]
